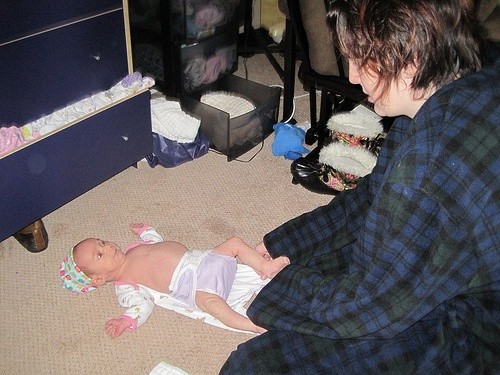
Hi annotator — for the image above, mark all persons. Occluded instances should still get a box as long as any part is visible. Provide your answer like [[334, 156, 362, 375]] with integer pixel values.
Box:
[[58, 221, 290, 339], [218, 0, 499, 375]]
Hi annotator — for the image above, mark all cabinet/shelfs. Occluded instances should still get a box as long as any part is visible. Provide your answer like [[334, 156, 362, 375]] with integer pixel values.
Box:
[[1, 0, 153, 244]]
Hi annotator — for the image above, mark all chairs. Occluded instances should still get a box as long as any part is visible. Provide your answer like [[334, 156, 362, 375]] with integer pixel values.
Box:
[[288, 0, 368, 147]]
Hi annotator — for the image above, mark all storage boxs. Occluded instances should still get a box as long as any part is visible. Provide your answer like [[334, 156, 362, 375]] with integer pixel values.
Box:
[[179, 75, 282, 162], [181, 26, 239, 95], [172, 0, 243, 45]]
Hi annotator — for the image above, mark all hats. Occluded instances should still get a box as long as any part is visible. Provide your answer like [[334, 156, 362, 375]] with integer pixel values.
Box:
[[59, 247, 96, 293]]
